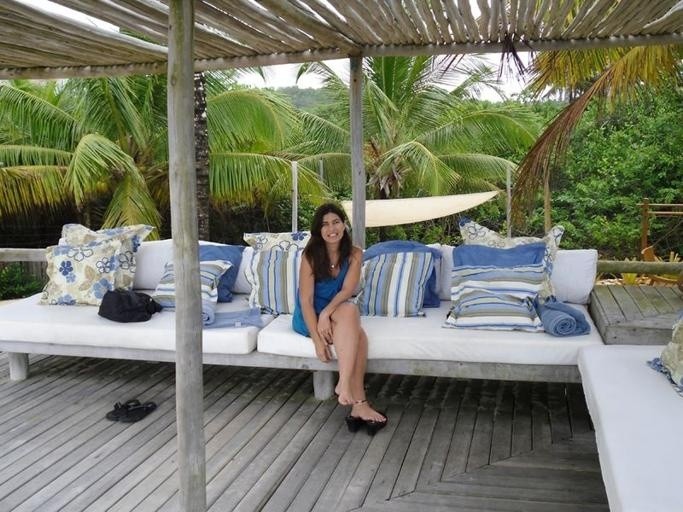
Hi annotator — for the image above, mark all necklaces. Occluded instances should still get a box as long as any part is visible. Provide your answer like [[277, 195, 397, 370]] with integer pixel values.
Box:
[[329, 263, 337, 269]]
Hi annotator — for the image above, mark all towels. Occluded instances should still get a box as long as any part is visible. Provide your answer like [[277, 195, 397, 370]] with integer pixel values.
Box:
[[540, 300, 590, 337], [200, 298, 263, 330]]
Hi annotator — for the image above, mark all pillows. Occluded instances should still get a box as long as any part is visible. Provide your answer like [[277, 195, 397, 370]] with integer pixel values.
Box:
[[36, 222, 311, 316], [646, 312, 682, 397], [353, 217, 564, 335]]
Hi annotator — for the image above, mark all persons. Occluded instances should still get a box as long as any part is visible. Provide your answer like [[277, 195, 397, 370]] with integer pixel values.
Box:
[[291, 202, 386, 424]]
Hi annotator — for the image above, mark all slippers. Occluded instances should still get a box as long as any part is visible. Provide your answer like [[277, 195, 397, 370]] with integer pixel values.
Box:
[[119, 401, 156, 423], [106, 399, 140, 421]]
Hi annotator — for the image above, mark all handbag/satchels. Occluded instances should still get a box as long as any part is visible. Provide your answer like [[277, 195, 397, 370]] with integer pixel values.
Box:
[[97, 286, 163, 322]]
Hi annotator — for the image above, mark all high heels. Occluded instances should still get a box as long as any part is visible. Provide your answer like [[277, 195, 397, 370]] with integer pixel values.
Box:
[[367, 411, 388, 436], [345, 415, 362, 433]]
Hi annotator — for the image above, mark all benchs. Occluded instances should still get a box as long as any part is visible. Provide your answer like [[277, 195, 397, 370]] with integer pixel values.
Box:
[[0, 238, 652, 399], [576, 345, 682, 512]]
